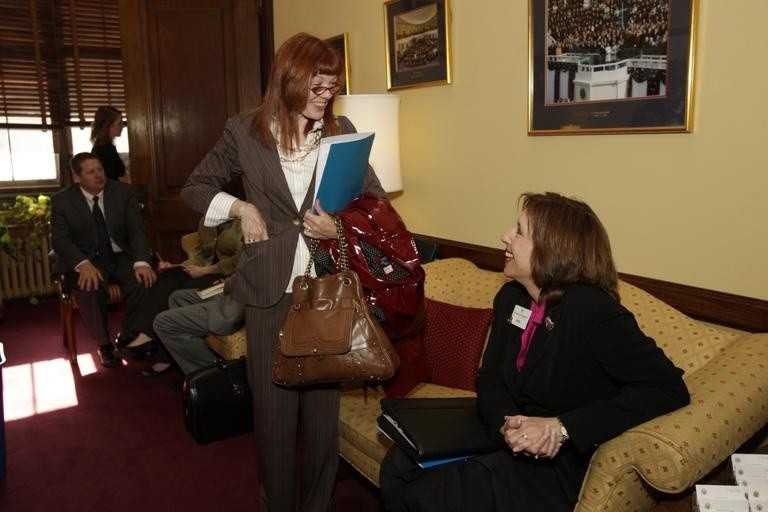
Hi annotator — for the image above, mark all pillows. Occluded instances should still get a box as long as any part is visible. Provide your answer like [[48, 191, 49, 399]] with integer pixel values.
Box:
[[424, 294, 495, 394]]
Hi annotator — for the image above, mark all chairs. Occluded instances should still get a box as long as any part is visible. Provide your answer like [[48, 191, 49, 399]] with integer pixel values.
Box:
[[52, 185, 164, 365]]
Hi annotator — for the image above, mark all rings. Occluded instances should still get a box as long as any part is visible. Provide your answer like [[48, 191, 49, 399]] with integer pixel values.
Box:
[[535, 454, 539, 459], [306, 226, 312, 233], [522, 434, 528, 440]]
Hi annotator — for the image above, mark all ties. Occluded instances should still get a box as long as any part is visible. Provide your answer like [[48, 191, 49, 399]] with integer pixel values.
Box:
[[93, 195, 115, 273]]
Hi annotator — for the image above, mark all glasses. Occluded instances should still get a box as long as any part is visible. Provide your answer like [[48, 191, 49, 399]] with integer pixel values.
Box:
[[308, 86, 339, 96]]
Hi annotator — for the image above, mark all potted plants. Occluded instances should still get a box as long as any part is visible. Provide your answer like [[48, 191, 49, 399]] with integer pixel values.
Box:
[[0, 192, 53, 305]]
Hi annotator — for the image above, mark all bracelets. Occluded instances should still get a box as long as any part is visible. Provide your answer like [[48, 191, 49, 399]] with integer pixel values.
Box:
[[555, 415, 572, 439]]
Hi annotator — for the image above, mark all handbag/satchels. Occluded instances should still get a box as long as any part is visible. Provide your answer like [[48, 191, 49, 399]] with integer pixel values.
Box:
[[274, 216, 400, 389], [184, 358, 256, 443]]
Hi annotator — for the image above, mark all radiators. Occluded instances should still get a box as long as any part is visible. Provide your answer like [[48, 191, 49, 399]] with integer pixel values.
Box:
[[1, 235, 56, 299]]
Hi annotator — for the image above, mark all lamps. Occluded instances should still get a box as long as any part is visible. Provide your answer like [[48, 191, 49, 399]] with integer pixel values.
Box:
[[338, 95, 404, 193]]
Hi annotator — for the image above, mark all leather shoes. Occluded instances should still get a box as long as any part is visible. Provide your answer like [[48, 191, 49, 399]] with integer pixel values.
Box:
[[98, 344, 121, 367]]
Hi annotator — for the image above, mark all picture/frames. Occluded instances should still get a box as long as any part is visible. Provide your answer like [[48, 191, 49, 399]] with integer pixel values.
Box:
[[382, 0, 452, 91], [318, 32, 351, 95], [527, 1, 700, 136]]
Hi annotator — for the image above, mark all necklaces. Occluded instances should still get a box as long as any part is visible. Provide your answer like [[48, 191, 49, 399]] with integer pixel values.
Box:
[[267, 128, 322, 163]]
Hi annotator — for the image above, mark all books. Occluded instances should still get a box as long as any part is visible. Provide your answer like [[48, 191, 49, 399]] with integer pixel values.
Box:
[[310, 130, 377, 217], [373, 384, 504, 474]]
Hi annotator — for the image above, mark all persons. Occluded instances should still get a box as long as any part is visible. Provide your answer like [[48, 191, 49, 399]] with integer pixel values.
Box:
[[48, 153, 160, 369], [378, 188, 690, 510], [91, 106, 125, 312], [181, 27, 388, 512], [549, 0, 667, 62]]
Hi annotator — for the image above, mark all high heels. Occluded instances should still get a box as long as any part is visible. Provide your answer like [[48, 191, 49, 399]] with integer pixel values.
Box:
[[141, 362, 172, 378], [114, 339, 161, 356]]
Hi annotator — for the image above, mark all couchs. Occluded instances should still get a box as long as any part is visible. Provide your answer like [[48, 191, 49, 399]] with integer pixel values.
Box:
[[338, 257, 768, 512]]
